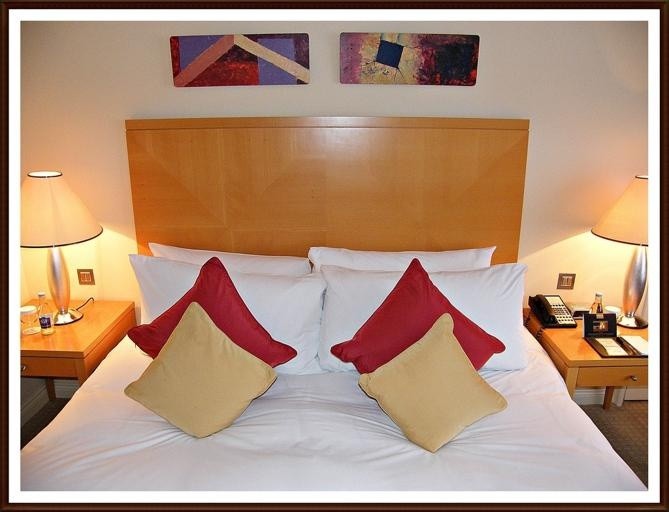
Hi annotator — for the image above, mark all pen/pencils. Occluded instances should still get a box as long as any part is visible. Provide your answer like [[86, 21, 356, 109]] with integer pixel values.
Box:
[[617, 336, 632, 354]]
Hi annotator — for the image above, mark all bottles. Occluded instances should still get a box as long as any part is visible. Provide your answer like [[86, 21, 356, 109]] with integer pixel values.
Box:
[[589, 292, 605, 314], [36, 292, 55, 335], [20, 306, 41, 334]]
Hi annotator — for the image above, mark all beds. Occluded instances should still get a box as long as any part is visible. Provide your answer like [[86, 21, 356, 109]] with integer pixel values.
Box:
[[21, 115, 647, 490]]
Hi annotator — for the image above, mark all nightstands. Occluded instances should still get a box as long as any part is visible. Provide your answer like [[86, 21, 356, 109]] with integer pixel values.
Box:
[[523, 305, 649, 410], [20, 298, 137, 401]]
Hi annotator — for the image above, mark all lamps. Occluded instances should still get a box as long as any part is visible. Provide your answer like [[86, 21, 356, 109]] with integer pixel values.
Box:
[[20, 169, 104, 326], [591, 173, 649, 330]]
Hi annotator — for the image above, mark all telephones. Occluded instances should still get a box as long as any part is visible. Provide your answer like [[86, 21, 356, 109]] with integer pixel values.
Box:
[[528, 294, 577, 328]]
